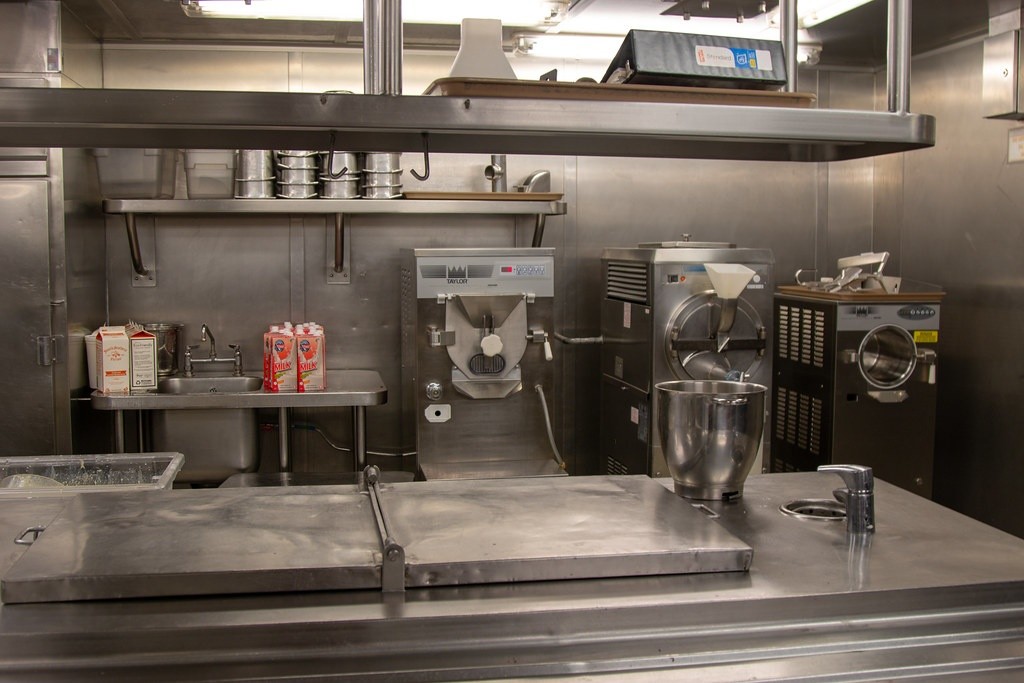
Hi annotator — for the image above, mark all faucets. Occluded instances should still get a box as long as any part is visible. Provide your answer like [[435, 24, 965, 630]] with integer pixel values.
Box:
[[200, 324, 217, 358], [817, 464, 875, 534]]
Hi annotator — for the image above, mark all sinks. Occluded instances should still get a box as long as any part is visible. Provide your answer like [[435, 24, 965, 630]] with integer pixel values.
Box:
[[780, 498, 848, 522], [146, 376, 264, 394]]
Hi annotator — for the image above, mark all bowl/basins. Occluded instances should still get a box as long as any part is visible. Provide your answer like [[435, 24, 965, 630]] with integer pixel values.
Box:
[[656, 379, 767, 501]]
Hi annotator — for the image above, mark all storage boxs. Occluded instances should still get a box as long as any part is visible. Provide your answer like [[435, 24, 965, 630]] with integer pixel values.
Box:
[[180, 148, 240, 200], [93, 148, 179, 200], [0, 452, 185, 498]]
